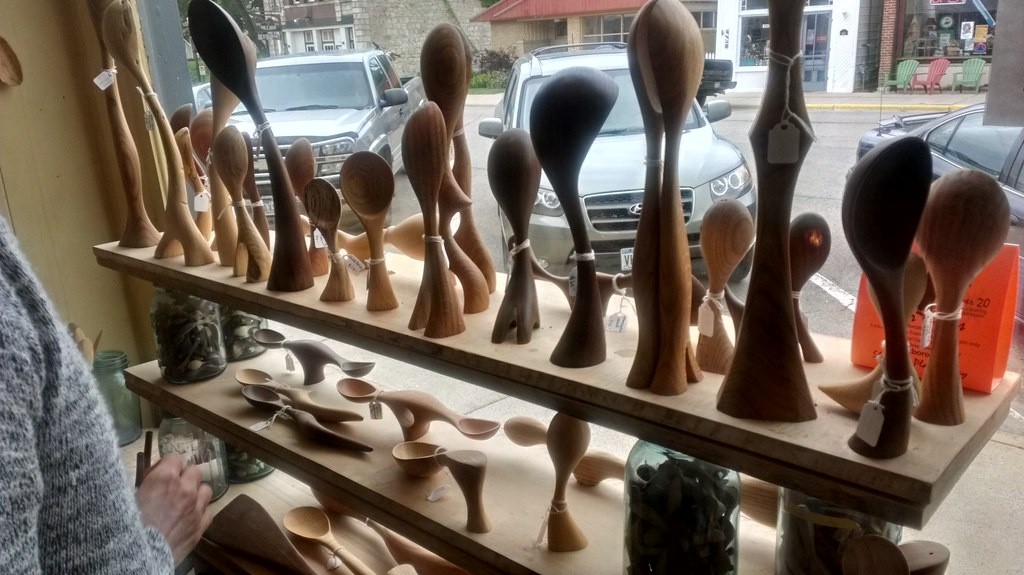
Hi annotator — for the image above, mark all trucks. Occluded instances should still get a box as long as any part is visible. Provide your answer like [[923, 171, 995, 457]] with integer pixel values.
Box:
[[228, 51, 430, 237]]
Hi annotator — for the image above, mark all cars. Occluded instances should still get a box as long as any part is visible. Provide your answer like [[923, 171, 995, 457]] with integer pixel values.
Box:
[[191, 81, 214, 114], [478, 39, 758, 288], [854, 102, 1023, 336]]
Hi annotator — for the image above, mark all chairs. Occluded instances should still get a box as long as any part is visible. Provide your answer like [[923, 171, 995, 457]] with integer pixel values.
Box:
[[951, 58, 987, 95], [884, 59, 920, 94], [910, 58, 951, 94]]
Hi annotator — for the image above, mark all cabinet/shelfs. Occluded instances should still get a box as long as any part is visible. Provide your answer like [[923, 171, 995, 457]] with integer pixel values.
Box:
[[93, 222, 1021, 575]]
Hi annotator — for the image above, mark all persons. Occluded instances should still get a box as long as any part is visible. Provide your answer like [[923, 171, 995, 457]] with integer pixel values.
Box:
[[0, 216, 214, 575]]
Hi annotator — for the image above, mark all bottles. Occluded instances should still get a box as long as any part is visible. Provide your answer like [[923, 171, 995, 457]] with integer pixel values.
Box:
[[91, 347, 142, 447], [157, 407, 276, 504]]
